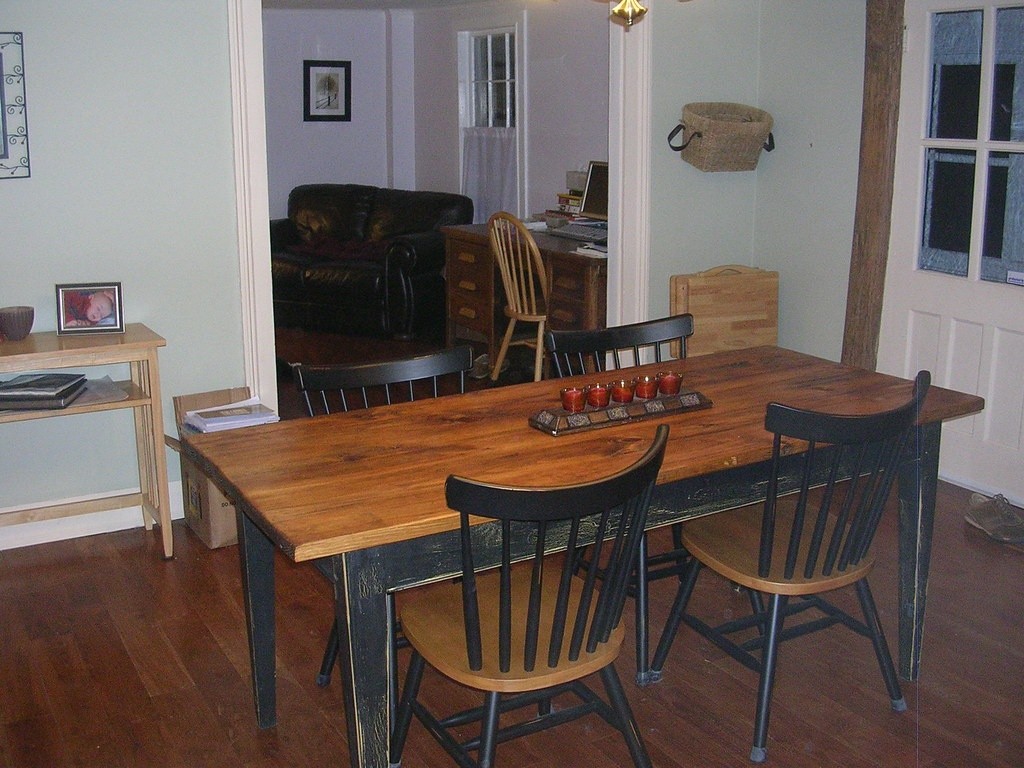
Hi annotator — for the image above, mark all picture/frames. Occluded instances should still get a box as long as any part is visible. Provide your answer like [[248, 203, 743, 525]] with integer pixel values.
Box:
[[55, 280, 126, 336], [301, 58, 353, 122], [0, 31, 31, 179]]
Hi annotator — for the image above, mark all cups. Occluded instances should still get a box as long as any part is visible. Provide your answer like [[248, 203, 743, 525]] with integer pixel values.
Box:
[[0, 304, 34, 340], [610, 379, 637, 403], [560, 386, 587, 412], [657, 371, 684, 395], [633, 375, 661, 398], [585, 383, 612, 407]]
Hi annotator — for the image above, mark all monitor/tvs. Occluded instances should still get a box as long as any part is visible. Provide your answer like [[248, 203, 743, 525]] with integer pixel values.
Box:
[[580, 160, 609, 230]]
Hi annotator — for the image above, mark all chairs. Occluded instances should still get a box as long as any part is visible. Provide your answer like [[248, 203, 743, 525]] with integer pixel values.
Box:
[[544, 313, 705, 601], [649, 370, 935, 761], [489, 211, 553, 380], [289, 340, 475, 714], [386, 418, 671, 767]]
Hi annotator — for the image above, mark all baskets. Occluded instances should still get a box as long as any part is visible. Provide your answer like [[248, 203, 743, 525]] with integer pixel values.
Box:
[[667, 102, 776, 173]]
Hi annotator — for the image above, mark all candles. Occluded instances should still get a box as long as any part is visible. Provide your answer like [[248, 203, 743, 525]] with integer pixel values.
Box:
[[636, 375, 662, 398], [561, 382, 587, 413], [612, 380, 634, 403], [588, 384, 611, 407], [659, 373, 682, 397]]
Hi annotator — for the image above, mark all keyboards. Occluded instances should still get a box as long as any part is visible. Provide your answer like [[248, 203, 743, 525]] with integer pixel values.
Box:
[[551, 224, 608, 243]]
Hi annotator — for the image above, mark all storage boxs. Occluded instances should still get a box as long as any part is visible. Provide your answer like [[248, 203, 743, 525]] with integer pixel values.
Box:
[[168, 387, 280, 552]]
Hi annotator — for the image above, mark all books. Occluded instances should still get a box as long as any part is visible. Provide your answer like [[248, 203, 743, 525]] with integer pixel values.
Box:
[[577, 236, 607, 258], [0, 374, 88, 410], [179, 395, 281, 436], [495, 217, 548, 231]]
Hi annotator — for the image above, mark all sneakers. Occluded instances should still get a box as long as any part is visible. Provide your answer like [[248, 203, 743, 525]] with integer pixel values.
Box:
[[965, 493, 1024, 552]]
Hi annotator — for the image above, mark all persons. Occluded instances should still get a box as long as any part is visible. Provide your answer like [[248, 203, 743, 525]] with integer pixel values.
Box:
[[64, 290, 115, 327]]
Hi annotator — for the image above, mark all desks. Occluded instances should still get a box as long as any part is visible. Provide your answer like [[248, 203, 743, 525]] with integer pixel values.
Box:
[[187, 340, 985, 766], [441, 214, 603, 373], [0, 325, 174, 563]]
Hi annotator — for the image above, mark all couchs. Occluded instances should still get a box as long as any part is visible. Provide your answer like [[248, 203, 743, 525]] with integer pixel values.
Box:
[[270, 184, 470, 342]]
